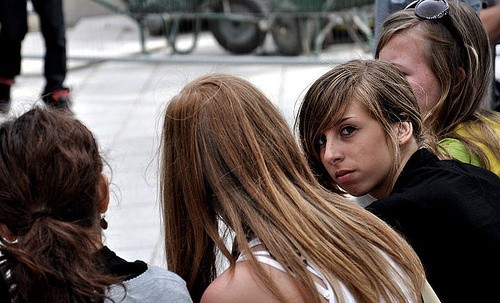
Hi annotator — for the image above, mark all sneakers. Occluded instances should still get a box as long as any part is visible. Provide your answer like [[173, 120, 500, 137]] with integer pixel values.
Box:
[[42, 87, 74, 117], [0, 79, 15, 113]]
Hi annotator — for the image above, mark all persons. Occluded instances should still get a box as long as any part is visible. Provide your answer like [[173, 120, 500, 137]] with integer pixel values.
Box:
[[0, 0, 73, 115], [0, 107, 192, 303], [375, 0, 500, 176], [294, 61, 500, 303], [159, 75, 441, 303]]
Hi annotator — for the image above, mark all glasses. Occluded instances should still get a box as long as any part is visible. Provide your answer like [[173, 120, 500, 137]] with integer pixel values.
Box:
[[403, 0, 470, 72]]
